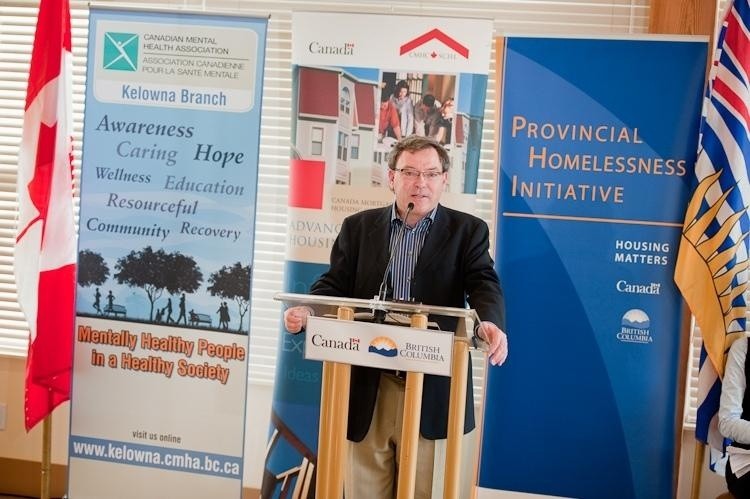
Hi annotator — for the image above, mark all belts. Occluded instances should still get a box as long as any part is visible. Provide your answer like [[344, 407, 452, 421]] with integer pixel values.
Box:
[[382, 369, 407, 379]]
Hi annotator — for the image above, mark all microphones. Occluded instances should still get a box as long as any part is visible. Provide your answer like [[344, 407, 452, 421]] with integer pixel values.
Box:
[[378, 203, 414, 300]]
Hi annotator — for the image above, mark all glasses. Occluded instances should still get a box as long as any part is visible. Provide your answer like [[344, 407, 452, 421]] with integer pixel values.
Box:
[[393, 168, 445, 181]]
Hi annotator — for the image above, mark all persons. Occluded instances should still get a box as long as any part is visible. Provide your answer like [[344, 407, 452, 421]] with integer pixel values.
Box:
[[283, 134, 508, 499], [717, 335, 749, 499], [378, 79, 456, 151]]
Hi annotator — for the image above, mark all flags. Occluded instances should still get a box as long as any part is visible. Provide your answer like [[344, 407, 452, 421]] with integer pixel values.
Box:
[[672, 0, 749, 447], [14, 0, 75, 436]]
